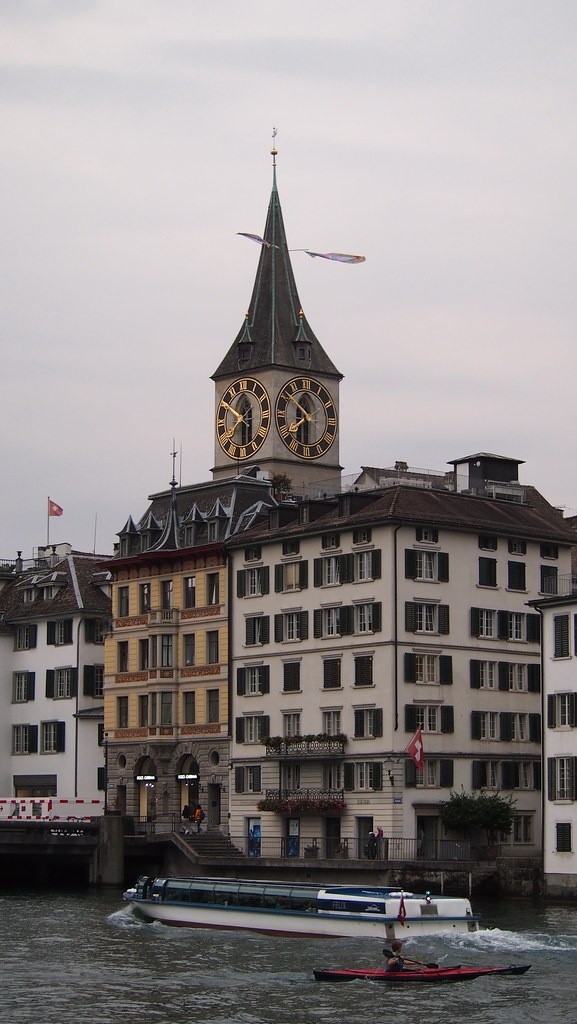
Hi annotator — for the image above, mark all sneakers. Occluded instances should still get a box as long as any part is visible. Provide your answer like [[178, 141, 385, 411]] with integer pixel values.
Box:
[[185, 830, 189, 835]]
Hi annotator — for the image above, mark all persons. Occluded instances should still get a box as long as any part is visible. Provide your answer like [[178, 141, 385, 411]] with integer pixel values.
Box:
[[192, 805, 204, 833], [181, 804, 192, 834], [367, 827, 383, 860], [32, 803, 42, 815], [12, 804, 18, 815], [385, 942, 424, 972]]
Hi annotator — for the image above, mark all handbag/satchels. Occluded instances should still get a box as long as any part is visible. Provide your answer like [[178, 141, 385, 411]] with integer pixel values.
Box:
[[200, 812, 205, 820]]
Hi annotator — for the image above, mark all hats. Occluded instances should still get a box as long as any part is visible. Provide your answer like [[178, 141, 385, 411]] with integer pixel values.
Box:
[[368, 832, 374, 835]]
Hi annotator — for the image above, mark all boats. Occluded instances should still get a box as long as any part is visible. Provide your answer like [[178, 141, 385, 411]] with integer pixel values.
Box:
[[313, 962, 531, 981], [122, 874, 481, 942]]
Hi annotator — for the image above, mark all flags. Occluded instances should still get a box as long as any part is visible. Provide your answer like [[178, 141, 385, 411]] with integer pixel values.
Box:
[[398, 899, 407, 926], [406, 729, 425, 774], [50, 501, 62, 516]]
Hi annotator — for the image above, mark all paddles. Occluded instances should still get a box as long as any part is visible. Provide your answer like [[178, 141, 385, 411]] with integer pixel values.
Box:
[[382, 948, 439, 969]]
